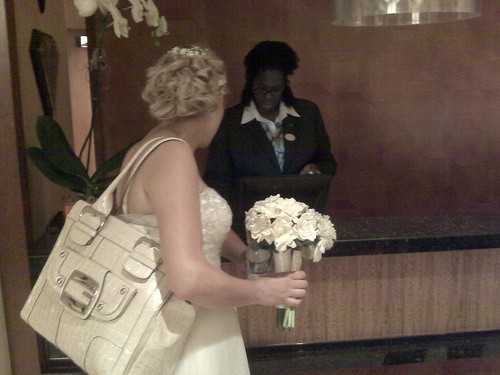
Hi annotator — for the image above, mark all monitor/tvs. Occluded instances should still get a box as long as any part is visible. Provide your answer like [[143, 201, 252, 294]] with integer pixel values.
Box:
[[238, 174, 332, 245]]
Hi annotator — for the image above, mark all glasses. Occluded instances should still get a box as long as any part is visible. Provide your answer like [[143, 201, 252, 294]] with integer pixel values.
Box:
[[253, 87, 282, 96]]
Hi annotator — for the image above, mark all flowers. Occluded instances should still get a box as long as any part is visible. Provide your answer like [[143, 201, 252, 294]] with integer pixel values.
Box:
[[250, 189, 337, 330], [26, 0, 169, 198]]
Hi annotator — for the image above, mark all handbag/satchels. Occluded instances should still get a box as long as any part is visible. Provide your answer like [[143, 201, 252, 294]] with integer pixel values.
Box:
[[20, 137, 196, 375]]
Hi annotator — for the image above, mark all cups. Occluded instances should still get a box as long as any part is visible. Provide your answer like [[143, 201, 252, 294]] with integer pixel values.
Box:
[[246, 249, 270, 278]]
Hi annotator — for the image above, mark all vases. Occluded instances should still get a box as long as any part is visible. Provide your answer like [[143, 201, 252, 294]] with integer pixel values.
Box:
[[64, 197, 93, 223]]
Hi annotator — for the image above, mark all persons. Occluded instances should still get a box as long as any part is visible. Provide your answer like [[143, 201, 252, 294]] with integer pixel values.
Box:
[[205, 41, 337, 224], [114, 46, 308, 375]]
